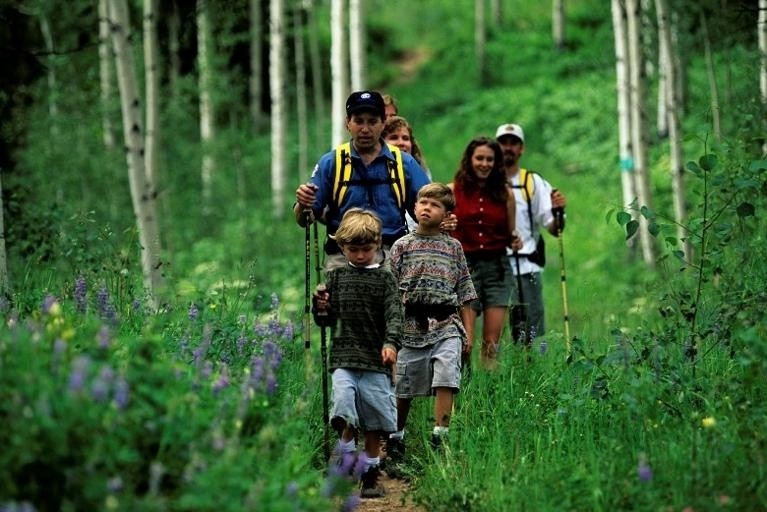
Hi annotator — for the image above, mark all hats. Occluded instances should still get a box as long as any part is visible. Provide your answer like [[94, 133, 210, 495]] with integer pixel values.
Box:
[[345, 89, 384, 119], [495, 123, 525, 144]]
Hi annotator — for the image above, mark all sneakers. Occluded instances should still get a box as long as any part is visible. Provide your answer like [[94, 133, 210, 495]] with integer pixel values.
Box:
[[328, 431, 450, 481], [360, 463, 384, 500]]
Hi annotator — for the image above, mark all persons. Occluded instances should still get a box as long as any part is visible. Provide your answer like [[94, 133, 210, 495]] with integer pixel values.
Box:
[[292, 90, 458, 268], [494, 124, 566, 363], [389, 183, 479, 454], [446, 137, 523, 368], [313, 209, 404, 497]]
